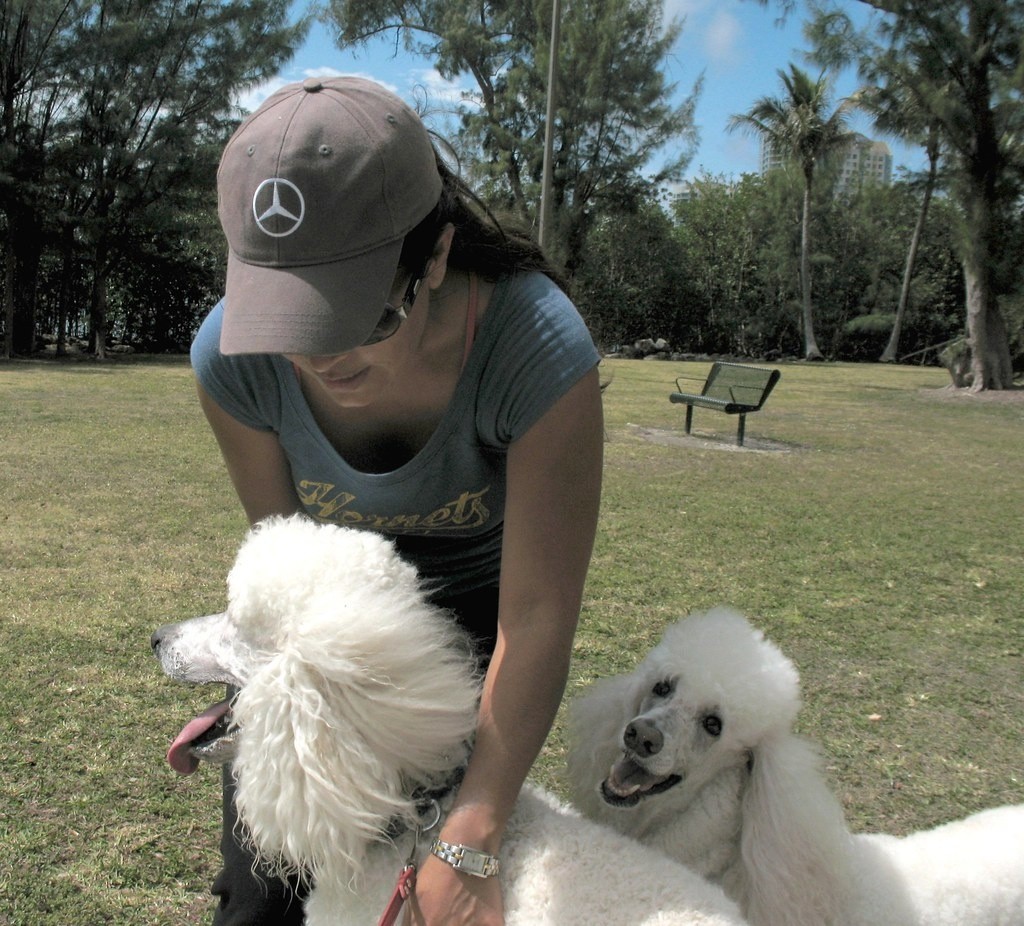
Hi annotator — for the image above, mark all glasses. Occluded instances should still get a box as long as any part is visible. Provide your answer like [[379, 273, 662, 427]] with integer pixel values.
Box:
[[367, 245, 439, 346]]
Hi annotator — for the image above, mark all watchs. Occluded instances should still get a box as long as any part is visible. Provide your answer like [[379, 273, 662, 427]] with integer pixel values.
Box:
[[429, 838, 501, 880]]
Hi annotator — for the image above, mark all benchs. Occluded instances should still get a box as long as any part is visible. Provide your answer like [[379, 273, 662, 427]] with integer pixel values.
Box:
[[669, 362, 780, 446]]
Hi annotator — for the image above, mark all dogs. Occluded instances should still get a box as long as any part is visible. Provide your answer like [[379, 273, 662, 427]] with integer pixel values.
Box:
[[157, 517, 750, 926], [574, 611, 1023, 926]]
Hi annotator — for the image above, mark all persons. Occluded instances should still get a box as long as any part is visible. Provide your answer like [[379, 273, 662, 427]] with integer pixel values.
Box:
[[184, 77, 602, 926]]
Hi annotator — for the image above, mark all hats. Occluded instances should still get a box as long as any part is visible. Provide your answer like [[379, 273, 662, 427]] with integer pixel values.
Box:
[[217, 78, 441, 357]]
